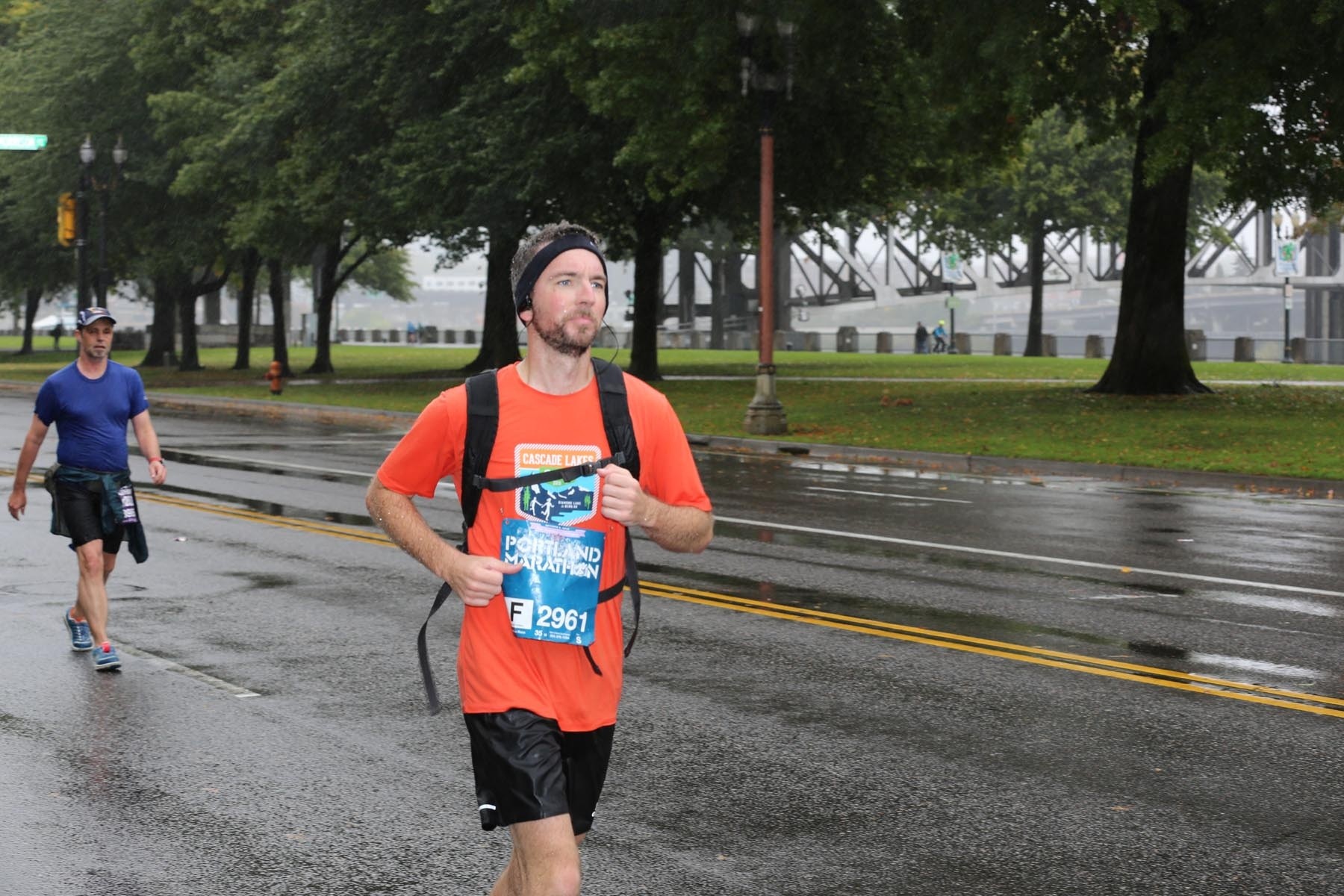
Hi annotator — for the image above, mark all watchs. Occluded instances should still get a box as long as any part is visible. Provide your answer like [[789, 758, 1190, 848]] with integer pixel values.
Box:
[[148, 456, 164, 466]]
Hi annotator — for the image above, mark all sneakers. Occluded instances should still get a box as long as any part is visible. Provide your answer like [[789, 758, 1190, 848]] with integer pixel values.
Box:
[[91, 641, 122, 670], [63, 607, 93, 651]]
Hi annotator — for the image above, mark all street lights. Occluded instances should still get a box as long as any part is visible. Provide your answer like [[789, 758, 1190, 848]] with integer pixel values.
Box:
[[75, 129, 127, 323], [740, 4, 812, 437]]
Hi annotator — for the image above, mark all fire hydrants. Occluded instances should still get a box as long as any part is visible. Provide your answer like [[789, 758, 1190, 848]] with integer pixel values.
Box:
[[262, 359, 285, 397]]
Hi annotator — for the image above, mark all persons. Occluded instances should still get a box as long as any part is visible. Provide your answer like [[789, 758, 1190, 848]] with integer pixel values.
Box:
[[7, 306, 166, 671], [406, 320, 428, 344], [48, 322, 63, 351], [931, 320, 948, 352], [915, 322, 929, 354], [362, 224, 712, 896]]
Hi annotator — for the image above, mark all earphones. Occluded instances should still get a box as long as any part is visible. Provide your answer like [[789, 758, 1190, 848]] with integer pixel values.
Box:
[[524, 296, 532, 307]]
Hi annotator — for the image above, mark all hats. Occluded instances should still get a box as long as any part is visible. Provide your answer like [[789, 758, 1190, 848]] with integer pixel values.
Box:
[[77, 307, 116, 325]]
